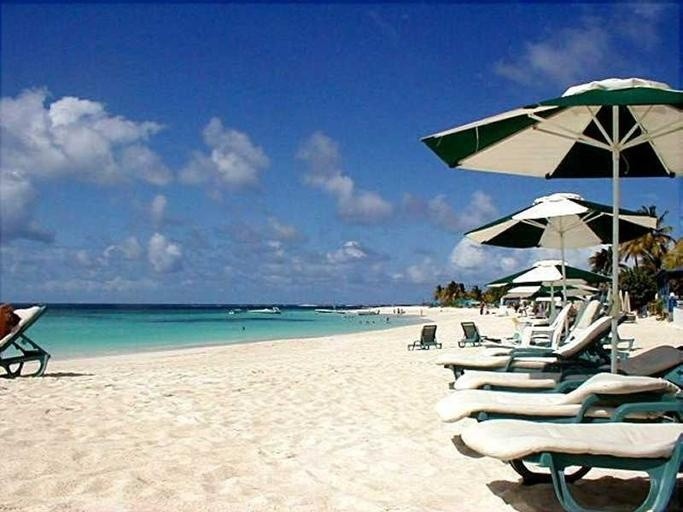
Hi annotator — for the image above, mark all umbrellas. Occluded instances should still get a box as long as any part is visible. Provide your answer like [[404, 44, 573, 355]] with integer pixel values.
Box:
[[464, 193, 657, 336], [421, 80, 683, 374], [484, 259, 612, 285], [504, 284, 597, 303]]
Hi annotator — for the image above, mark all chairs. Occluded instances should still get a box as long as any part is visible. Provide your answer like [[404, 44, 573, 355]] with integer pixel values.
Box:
[[0, 304, 52, 378], [407, 324, 443, 351], [436, 300, 682, 511]]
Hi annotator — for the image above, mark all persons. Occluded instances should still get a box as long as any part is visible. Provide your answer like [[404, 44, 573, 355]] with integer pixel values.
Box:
[[0, 303, 20, 339]]
